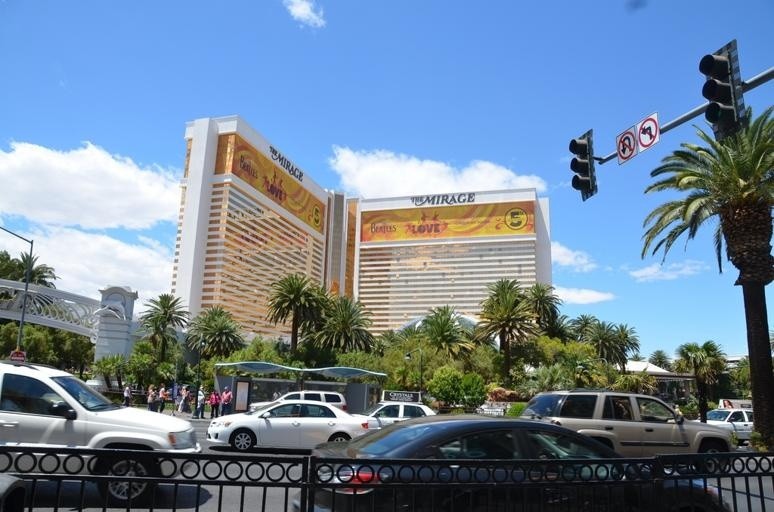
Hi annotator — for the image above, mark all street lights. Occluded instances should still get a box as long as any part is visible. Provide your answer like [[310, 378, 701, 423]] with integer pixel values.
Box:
[[578, 359, 610, 394], [405, 348, 423, 401]]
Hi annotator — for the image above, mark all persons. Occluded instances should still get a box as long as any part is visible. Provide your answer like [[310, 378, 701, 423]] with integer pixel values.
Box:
[[123, 381, 233, 419], [723, 399, 733, 411], [1, 379, 51, 414], [254, 386, 294, 402], [290, 406, 299, 416], [675, 404, 685, 415]]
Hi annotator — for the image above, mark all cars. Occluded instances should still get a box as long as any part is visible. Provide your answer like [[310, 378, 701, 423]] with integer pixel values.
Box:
[[431, 400, 450, 412], [353, 390, 436, 428], [290, 416, 732, 511], [685, 398, 755, 443], [205, 400, 381, 453]]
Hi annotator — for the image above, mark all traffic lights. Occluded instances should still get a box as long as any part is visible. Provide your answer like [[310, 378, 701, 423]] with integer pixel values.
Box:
[[568, 128, 592, 194]]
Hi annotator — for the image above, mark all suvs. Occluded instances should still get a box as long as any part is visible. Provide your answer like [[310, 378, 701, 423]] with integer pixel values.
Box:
[[520, 391, 738, 475], [250, 391, 347, 416], [1, 361, 200, 504]]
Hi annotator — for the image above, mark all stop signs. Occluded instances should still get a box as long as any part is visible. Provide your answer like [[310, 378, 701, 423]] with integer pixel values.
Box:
[[8, 350, 26, 364]]
